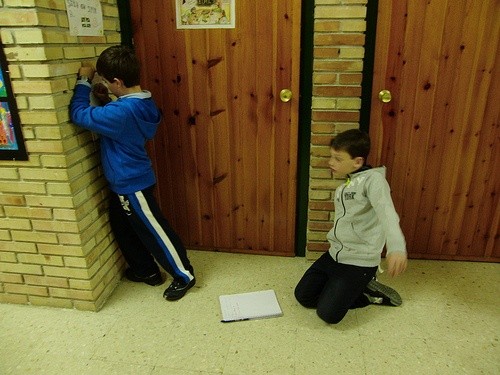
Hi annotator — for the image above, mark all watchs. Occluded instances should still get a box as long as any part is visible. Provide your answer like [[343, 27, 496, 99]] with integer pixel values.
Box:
[[77, 75, 92, 85]]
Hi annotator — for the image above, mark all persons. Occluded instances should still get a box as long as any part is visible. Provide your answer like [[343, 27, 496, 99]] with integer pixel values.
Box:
[[294, 129, 408, 324], [69, 45, 196, 300]]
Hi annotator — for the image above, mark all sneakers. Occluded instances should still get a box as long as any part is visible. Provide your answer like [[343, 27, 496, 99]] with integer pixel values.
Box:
[[364, 278, 401, 306], [122, 264, 163, 284], [164, 271, 196, 301]]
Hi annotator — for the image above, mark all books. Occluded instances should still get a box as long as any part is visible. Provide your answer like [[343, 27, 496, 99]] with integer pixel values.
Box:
[[85, 80, 120, 142], [219, 289, 283, 321]]
